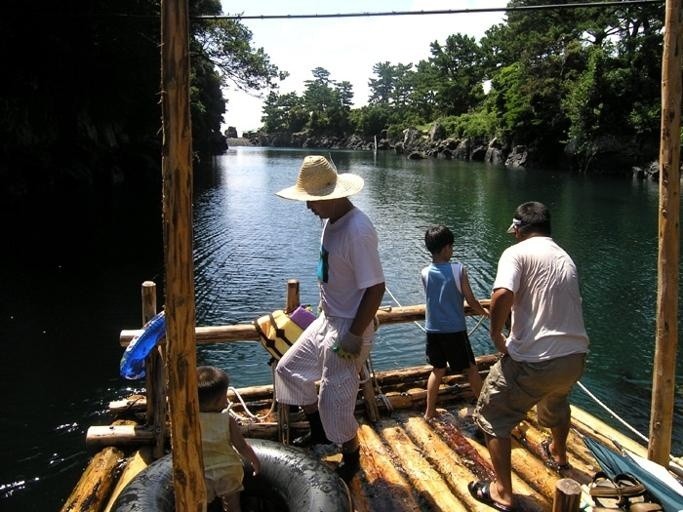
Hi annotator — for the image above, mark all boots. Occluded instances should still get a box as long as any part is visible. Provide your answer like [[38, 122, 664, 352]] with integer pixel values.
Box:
[[334, 446, 361, 483], [293, 411, 333, 447]]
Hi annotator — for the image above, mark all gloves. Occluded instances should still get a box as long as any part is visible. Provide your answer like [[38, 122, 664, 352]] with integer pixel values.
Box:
[[338, 331, 362, 359]]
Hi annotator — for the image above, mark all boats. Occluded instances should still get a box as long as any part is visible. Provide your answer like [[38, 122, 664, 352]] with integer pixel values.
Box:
[[61, 0, 683, 511]]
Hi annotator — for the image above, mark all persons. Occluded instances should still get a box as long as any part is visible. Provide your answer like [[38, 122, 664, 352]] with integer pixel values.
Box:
[[423, 226, 490, 421], [468, 203, 586, 512], [195, 365, 260, 512], [276, 156, 386, 482]]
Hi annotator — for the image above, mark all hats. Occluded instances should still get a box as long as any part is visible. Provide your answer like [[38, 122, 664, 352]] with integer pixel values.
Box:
[[506, 218, 521, 233], [275, 155, 364, 201]]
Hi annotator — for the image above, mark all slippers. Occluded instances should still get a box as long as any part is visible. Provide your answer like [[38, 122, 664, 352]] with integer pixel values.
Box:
[[540, 440, 571, 471], [468, 479, 512, 512]]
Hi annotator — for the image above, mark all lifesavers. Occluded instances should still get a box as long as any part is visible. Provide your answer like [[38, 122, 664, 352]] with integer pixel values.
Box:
[[120, 310, 166, 379]]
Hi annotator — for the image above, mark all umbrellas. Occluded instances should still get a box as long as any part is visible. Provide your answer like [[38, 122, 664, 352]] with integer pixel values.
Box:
[[572, 427, 683, 512]]
